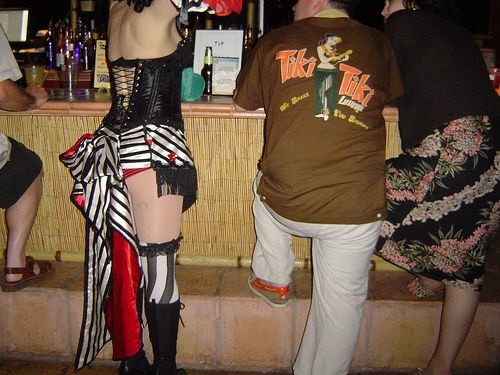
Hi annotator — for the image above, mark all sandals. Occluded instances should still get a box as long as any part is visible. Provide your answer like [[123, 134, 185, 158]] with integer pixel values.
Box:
[[1, 254, 56, 292]]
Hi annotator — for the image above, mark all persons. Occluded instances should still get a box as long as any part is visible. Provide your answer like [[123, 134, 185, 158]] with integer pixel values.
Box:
[[58, 0, 242, 375], [371, 0, 500, 375], [231, 0, 405, 375], [0, 22, 54, 292]]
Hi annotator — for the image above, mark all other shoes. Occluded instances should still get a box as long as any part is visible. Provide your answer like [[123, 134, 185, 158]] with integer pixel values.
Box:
[[248, 274, 289, 308]]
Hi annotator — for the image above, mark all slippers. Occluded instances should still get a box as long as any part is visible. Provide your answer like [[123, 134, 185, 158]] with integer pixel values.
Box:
[[411, 277, 446, 298]]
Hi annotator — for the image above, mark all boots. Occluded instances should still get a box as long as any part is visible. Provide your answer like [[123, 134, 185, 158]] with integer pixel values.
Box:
[[144, 296, 185, 375], [118, 287, 151, 375]]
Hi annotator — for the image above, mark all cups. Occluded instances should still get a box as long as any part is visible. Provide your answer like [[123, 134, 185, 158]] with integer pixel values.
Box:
[[60, 64, 80, 101], [24, 67, 43, 86]]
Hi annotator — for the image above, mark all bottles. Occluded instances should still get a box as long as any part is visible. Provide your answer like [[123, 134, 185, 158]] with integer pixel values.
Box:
[[44, 17, 99, 70], [201, 46, 213, 95], [179, 15, 253, 50]]
